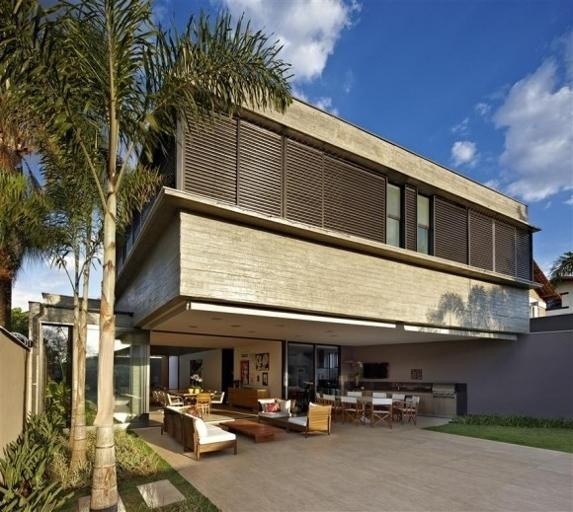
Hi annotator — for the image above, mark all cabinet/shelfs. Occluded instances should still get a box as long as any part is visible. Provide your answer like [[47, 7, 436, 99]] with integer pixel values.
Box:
[[227, 386, 268, 415]]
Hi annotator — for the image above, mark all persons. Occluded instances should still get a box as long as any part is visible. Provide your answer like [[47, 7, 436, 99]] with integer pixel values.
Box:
[[239, 363, 248, 385], [255, 354, 261, 371], [262, 354, 269, 372]]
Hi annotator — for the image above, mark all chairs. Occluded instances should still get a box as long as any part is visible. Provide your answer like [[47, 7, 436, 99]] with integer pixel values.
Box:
[[151, 388, 224, 416], [160, 406, 237, 461], [315, 390, 420, 430], [258, 397, 332, 438]]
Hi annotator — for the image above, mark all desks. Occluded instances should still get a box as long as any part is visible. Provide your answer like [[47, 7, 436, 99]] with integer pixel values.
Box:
[[219, 419, 283, 442]]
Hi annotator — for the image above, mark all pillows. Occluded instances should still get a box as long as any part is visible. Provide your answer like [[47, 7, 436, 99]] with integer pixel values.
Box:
[[186, 412, 209, 438], [277, 398, 292, 415], [164, 405, 182, 415], [263, 402, 281, 412], [257, 398, 276, 407]]
